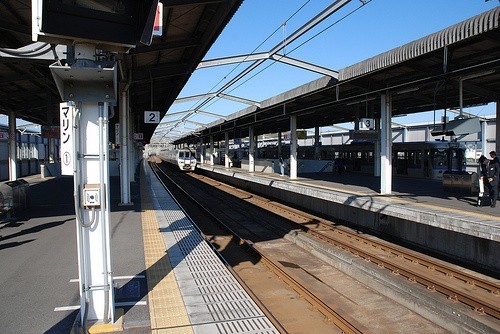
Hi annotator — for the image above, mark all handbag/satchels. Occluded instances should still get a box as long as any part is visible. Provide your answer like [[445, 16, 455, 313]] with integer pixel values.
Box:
[[478, 193, 492, 205]]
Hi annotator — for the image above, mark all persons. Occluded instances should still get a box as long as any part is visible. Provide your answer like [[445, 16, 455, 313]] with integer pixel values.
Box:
[[477, 155, 498, 207], [489, 151, 500, 201], [287, 155, 290, 174], [278, 155, 285, 177], [335, 153, 353, 178]]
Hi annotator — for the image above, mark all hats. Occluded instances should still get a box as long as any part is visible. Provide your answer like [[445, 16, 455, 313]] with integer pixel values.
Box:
[[479, 156, 486, 163]]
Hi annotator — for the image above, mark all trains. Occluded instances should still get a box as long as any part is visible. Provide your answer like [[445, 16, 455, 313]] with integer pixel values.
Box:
[[200, 140, 468, 180], [177, 149, 198, 171]]
